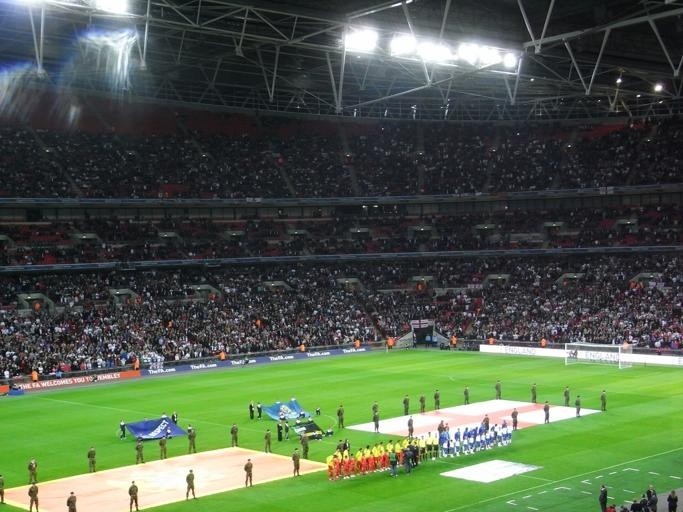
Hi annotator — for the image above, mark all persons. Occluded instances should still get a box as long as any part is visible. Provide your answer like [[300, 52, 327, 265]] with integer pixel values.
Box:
[[0, 422, 309, 511], [1, 116, 683, 390], [666, 489, 678, 511], [326, 381, 607, 481], [249, 399, 262, 420], [279, 406, 321, 420], [598, 484, 658, 511], [120, 411, 179, 441], [313, 431, 318, 440]]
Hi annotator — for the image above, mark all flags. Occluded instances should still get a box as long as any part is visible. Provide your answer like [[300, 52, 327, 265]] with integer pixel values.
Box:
[[265, 399, 313, 420], [125, 416, 188, 440]]
